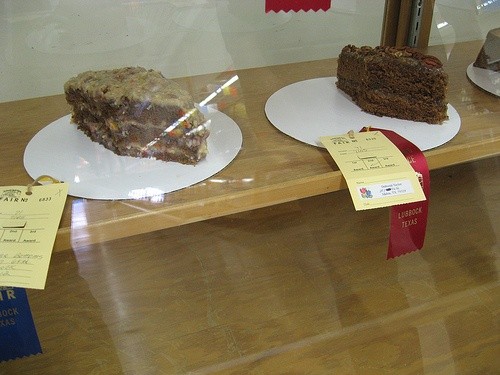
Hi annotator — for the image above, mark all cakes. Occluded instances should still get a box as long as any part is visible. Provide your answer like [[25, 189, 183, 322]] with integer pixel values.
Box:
[[472, 28, 500, 73], [334, 45, 450, 123], [63, 66, 208, 165]]
